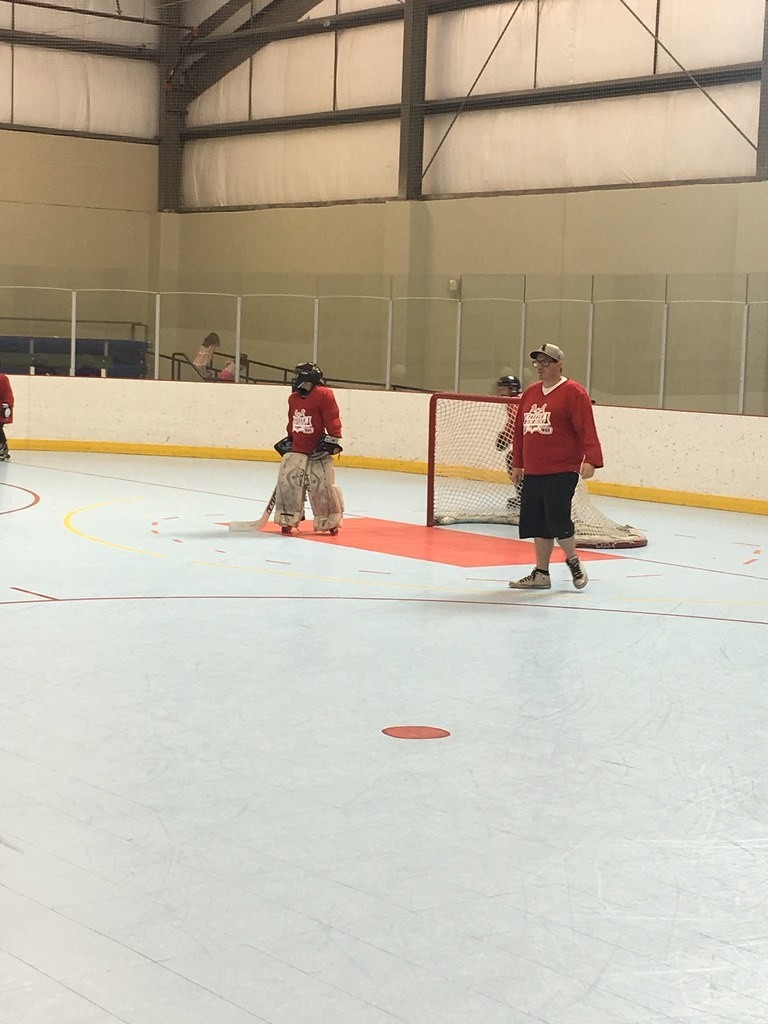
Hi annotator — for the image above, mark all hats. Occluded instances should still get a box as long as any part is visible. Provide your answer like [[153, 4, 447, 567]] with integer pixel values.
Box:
[[529, 344, 565, 362]]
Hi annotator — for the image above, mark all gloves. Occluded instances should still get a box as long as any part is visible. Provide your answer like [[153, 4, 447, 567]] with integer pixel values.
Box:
[[273, 436, 292, 456], [313, 435, 343, 455], [496, 434, 511, 453], [2, 403, 12, 418]]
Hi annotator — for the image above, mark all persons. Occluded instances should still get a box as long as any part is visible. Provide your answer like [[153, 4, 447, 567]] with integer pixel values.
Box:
[[0, 373, 14, 462], [509, 343, 604, 589], [496, 375, 524, 507], [193, 333, 247, 382], [274, 362, 345, 536]]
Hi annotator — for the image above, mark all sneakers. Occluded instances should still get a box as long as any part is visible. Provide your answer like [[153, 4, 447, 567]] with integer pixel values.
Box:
[[0, 444, 10, 461], [509, 570, 551, 588], [565, 554, 588, 589], [507, 496, 523, 510]]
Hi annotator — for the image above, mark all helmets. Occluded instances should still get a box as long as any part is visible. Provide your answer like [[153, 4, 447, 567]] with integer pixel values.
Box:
[[292, 362, 323, 393], [497, 375, 520, 396]]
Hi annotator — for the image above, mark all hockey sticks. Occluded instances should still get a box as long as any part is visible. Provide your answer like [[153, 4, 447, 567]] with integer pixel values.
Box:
[[229, 485, 277, 532]]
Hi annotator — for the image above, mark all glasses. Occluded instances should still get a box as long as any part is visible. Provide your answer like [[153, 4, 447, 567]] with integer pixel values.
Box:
[[532, 359, 557, 367]]
